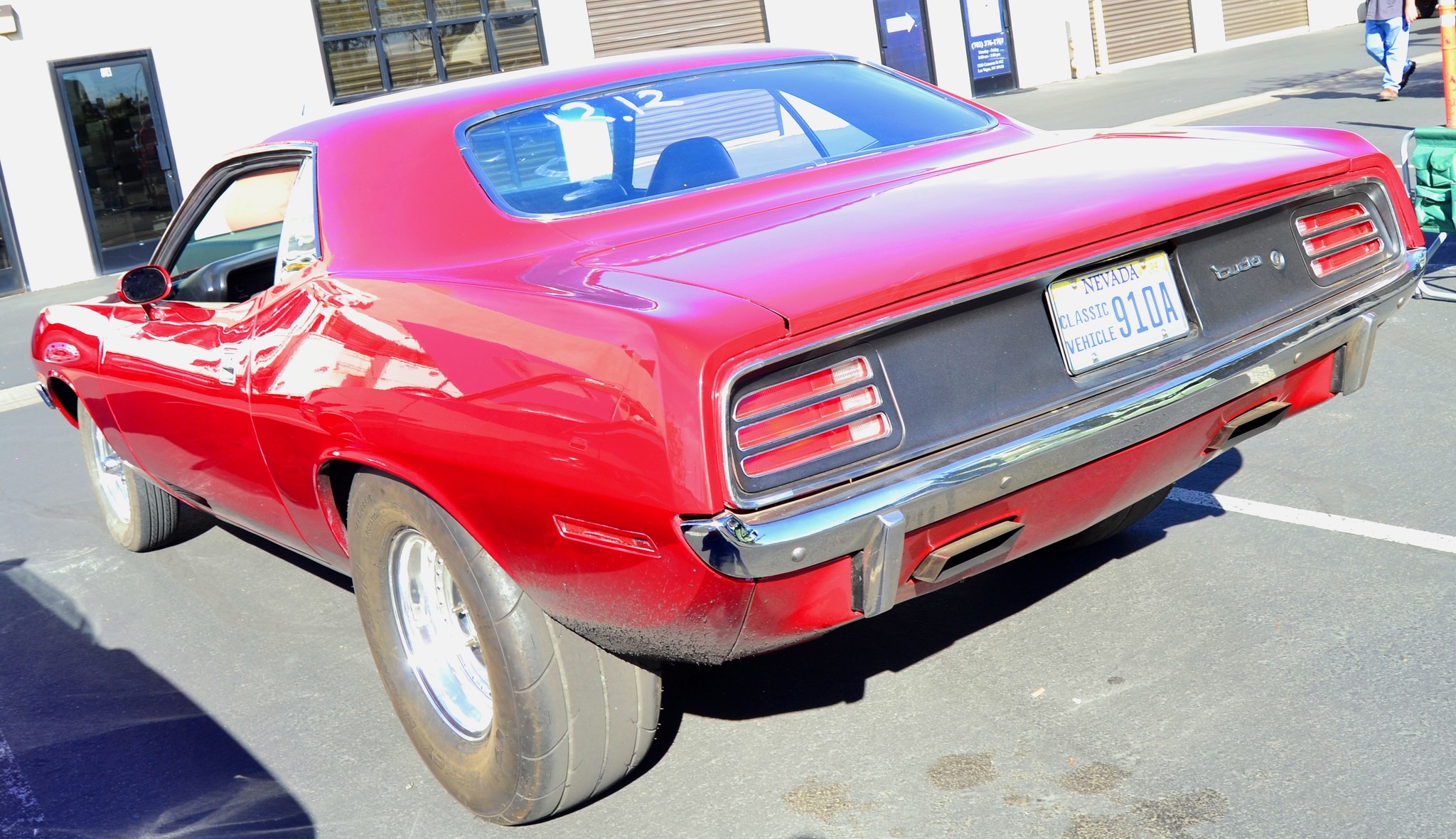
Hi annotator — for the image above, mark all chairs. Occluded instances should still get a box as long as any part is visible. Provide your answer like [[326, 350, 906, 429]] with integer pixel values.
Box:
[[1401, 127, 1456, 301], [643, 136, 739, 198]]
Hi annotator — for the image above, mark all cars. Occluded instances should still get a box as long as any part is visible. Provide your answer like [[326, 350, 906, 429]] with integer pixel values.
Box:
[[32, 40, 1427, 827]]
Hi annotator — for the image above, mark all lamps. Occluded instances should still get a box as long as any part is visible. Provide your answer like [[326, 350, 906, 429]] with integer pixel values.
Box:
[[0, 5, 17, 35]]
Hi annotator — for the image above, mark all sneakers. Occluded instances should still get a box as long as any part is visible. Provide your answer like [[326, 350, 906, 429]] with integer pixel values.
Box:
[[1379, 89, 1397, 100], [1399, 61, 1416, 90]]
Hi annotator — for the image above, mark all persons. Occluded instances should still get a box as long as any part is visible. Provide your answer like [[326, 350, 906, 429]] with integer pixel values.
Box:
[[81, 159, 123, 212], [131, 118, 157, 169], [1364, 0, 1418, 101]]
